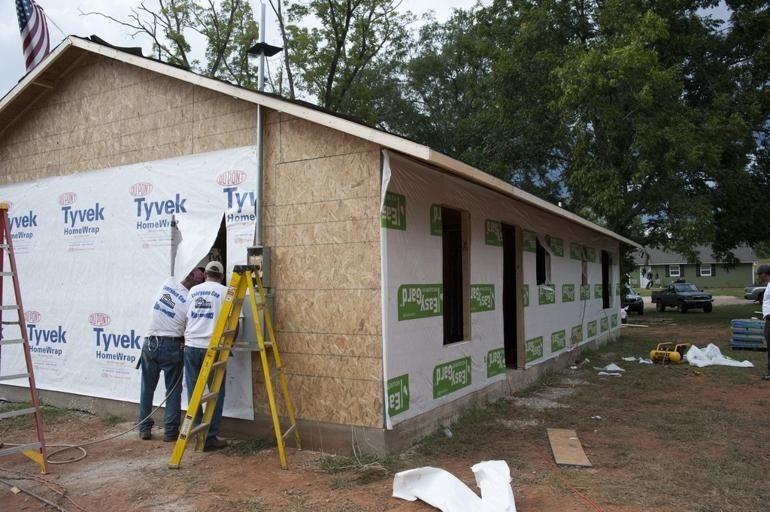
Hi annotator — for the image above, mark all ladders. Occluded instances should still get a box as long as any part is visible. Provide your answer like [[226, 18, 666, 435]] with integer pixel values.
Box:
[[0, 203, 49, 474], [169, 264, 302, 470]]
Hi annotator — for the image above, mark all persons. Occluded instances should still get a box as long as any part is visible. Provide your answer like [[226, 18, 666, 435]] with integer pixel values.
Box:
[[139, 270, 204, 442], [755, 265, 770, 380], [184, 261, 239, 452]]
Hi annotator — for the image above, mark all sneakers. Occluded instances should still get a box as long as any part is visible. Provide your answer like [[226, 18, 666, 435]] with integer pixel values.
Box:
[[205, 440, 226, 451], [139, 432, 151, 440], [164, 431, 179, 442]]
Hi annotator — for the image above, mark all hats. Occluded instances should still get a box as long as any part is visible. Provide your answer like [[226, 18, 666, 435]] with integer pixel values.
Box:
[[205, 261, 224, 273], [757, 265, 770, 274]]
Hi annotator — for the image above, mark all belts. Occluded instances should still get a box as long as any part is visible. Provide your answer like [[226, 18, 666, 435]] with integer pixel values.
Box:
[[152, 337, 181, 341]]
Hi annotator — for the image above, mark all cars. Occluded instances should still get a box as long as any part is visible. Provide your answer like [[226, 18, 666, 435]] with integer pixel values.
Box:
[[621, 285, 644, 315]]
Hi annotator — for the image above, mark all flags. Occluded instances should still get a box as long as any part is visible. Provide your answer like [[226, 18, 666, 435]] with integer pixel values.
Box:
[[15, 0, 50, 71]]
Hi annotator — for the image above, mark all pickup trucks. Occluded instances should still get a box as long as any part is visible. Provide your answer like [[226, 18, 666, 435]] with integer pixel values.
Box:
[[651, 283, 714, 313], [743, 279, 768, 304]]
